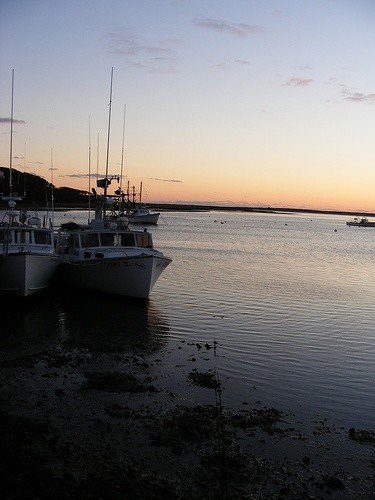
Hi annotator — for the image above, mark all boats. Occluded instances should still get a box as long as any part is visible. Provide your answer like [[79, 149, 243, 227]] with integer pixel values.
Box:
[[345, 217, 375, 228], [117, 181, 161, 224], [0, 66, 64, 301], [56, 65, 173, 301]]
